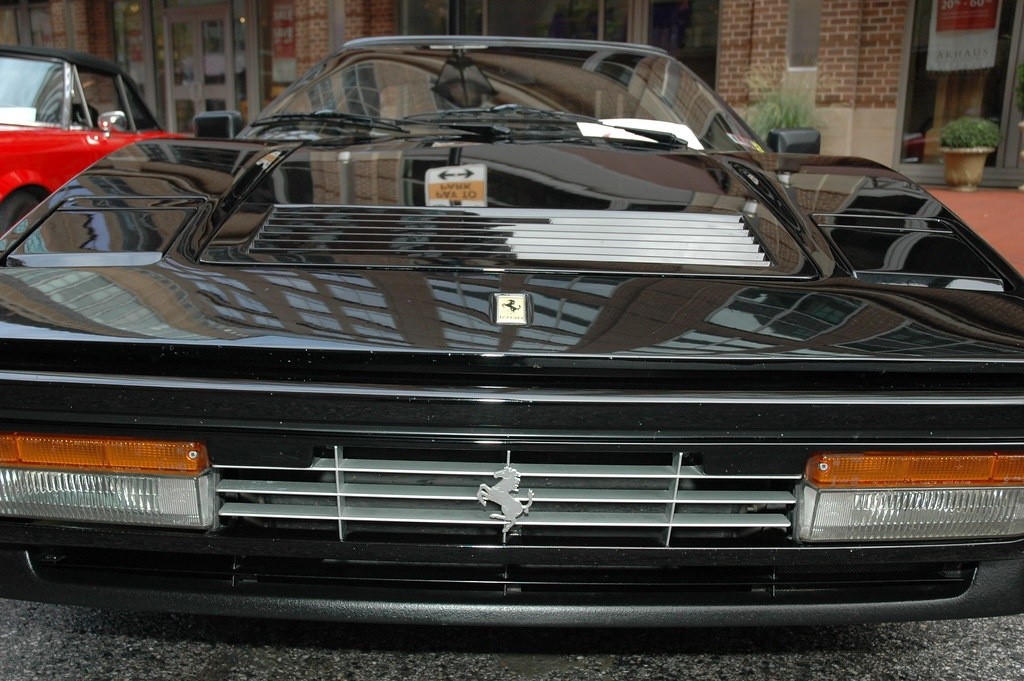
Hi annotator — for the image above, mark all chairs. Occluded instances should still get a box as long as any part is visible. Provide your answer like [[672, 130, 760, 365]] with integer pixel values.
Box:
[[70, 103, 98, 130]]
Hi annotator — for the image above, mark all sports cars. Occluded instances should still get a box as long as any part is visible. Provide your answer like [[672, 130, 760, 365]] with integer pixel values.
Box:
[[2, 32, 1024, 627], [0, 46, 194, 238]]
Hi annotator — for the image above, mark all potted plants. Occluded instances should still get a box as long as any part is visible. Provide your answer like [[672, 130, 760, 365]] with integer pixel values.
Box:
[[939, 118, 1001, 191], [1015, 64, 1024, 190]]
